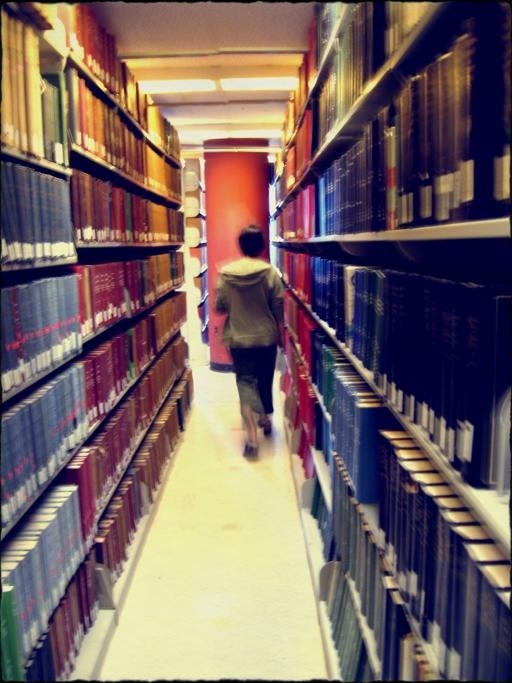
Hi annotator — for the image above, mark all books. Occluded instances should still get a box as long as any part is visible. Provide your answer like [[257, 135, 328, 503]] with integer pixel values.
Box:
[[1, 250, 185, 394], [1, 335, 192, 682], [1, 290, 185, 539], [268, 0, 510, 682], [3, 162, 184, 264], [2, 0, 183, 208]]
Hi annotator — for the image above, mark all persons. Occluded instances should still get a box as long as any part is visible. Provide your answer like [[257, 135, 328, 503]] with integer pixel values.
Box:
[[210, 222, 285, 460]]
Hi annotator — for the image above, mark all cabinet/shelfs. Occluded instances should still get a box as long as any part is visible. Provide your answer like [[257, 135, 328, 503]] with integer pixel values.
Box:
[[271, 0, 512, 681], [0, 1, 211, 682]]
